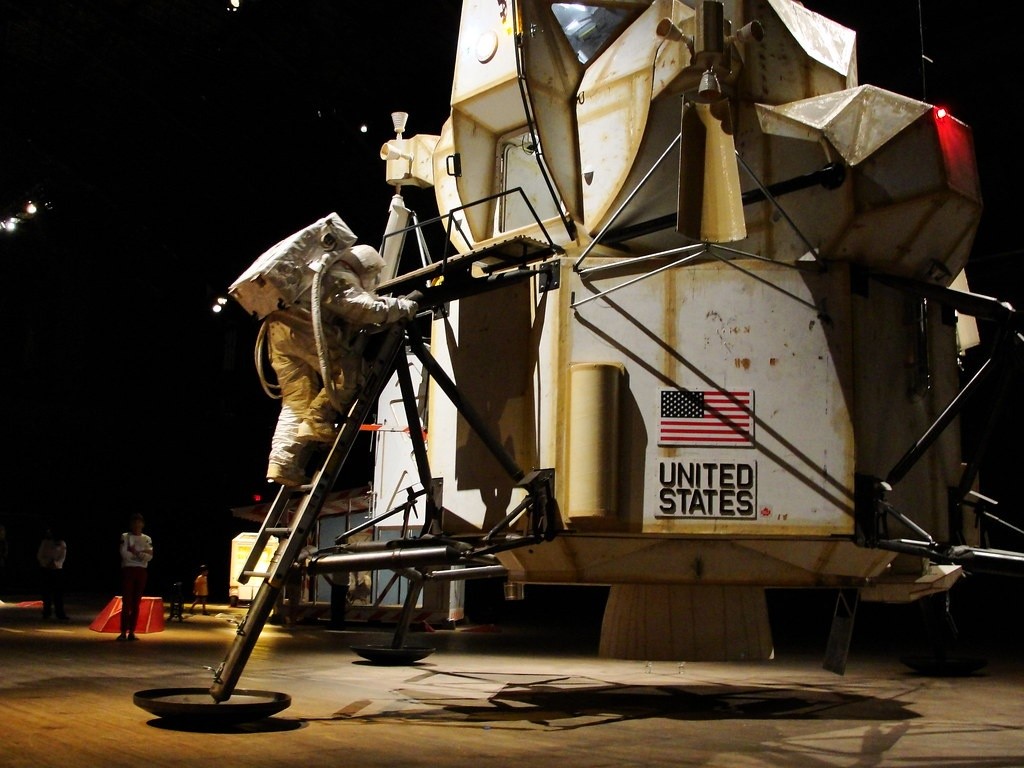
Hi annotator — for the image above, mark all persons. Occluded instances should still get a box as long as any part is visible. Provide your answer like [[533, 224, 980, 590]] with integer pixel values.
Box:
[[189, 564, 211, 615], [264, 244, 418, 486], [116, 515, 153, 640], [36, 525, 70, 622]]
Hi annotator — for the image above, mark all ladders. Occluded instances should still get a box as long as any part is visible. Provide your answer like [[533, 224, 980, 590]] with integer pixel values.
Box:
[[201, 289, 423, 706]]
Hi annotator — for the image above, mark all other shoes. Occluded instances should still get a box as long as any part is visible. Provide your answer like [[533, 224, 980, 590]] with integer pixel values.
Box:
[[117, 635, 127, 639], [58, 615, 70, 620], [128, 634, 139, 641], [42, 613, 50, 618], [296, 423, 335, 442], [189, 609, 196, 615], [265, 464, 306, 486], [202, 611, 211, 615]]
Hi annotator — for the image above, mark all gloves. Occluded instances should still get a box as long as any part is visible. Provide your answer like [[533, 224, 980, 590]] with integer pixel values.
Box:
[[376, 296, 419, 324]]
